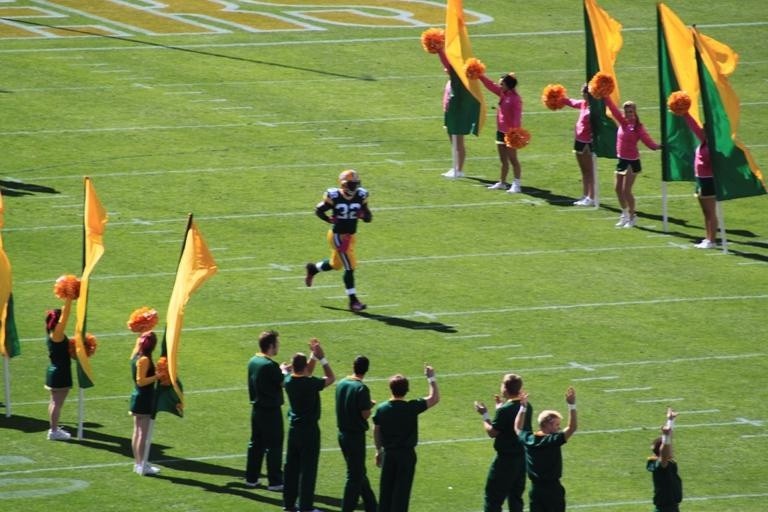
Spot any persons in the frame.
[45,309,72,440]
[602,95,664,227]
[513,386,578,511]
[305,170,374,311]
[669,105,720,249]
[245,329,292,491]
[474,373,534,512]
[281,337,336,512]
[372,361,440,512]
[560,84,596,208]
[646,406,682,512]
[334,355,377,512]
[436,48,465,178]
[128,331,165,475]
[476,71,523,193]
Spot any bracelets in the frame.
[319,358,328,367]
[309,353,318,361]
[665,419,674,429]
[482,413,489,421]
[427,376,436,383]
[567,403,577,410]
[520,406,527,413]
[661,435,673,445]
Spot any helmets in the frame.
[337,169,360,187]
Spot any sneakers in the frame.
[132,459,161,476]
[266,482,284,491]
[573,193,594,207]
[304,263,315,287]
[46,424,73,442]
[615,210,639,229]
[346,300,367,310]
[245,476,262,487]
[694,236,718,250]
[505,181,523,193]
[441,167,465,178]
[488,180,507,191]
